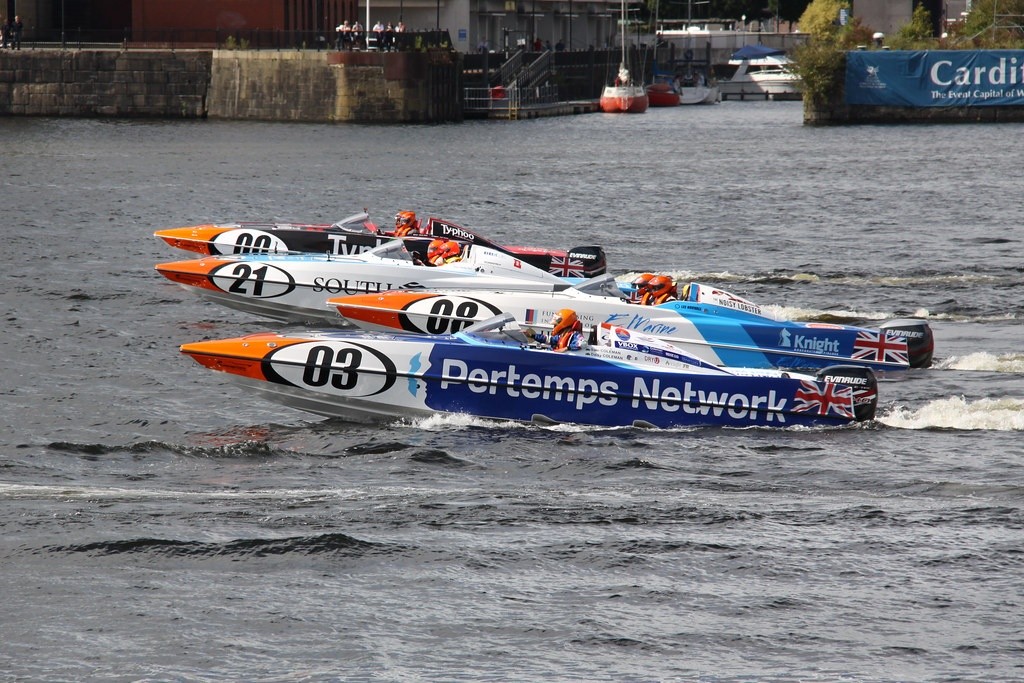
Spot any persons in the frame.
[385,21,393,52]
[336,20,350,51]
[395,21,406,52]
[377,211,419,238]
[352,20,363,52]
[414,31,709,95]
[10,16,23,49]
[623,273,675,306]
[0,19,9,49]
[520,309,588,353]
[412,240,462,266]
[373,21,384,53]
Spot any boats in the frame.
[150,211,937,435]
[716,42,807,101]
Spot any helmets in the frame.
[396,211,405,227]
[401,212,415,227]
[633,274,655,294]
[649,275,671,296]
[440,242,460,258]
[427,240,444,257]
[550,309,576,335]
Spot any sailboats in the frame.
[598,0,720,113]
